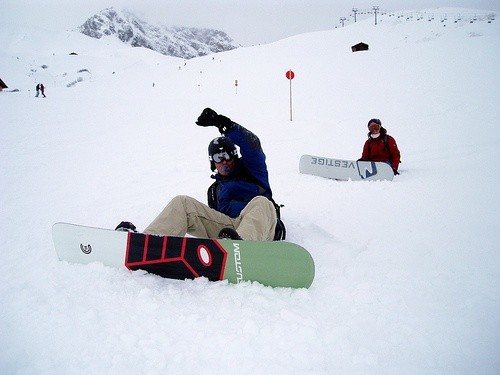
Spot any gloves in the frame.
[196,108,232,134]
[393,169,399,175]
[357,158,364,161]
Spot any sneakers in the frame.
[217,228,243,240]
[114,221,137,232]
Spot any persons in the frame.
[35,83,41,97]
[41,84,46,96]
[114,106,287,241]
[357,118,401,177]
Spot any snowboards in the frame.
[51,222,316,289]
[299,154,394,181]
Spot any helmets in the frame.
[208,137,238,172]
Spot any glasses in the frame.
[369,125,379,132]
[209,148,238,163]
